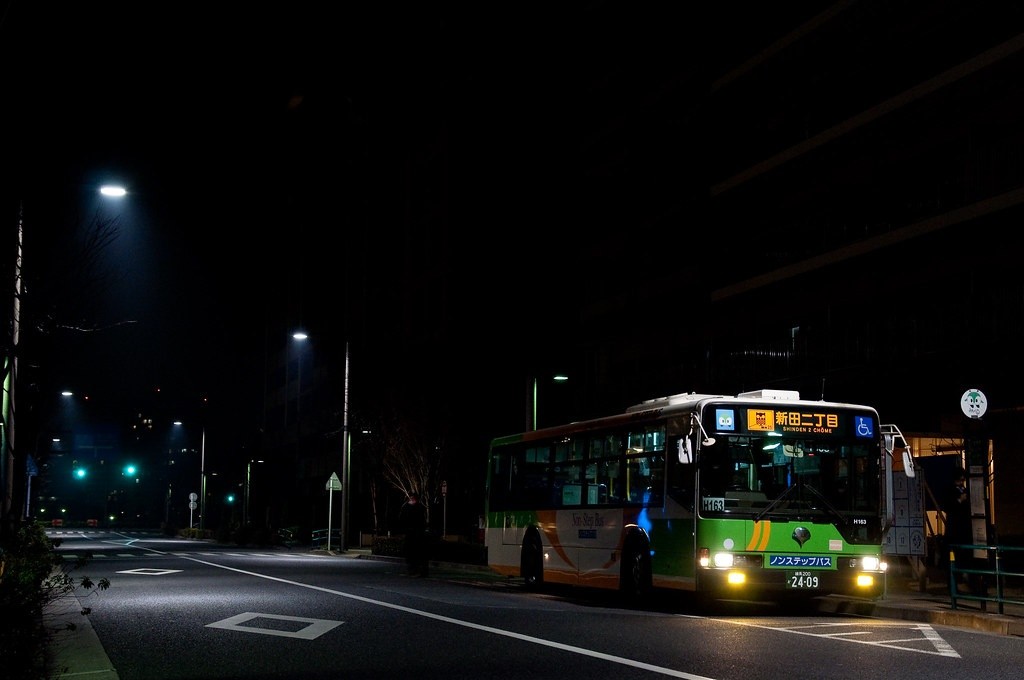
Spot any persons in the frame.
[945,467,970,594]
[398,491,432,579]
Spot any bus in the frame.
[484,389,915,617]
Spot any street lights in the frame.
[533,374,569,432]
[174,420,206,530]
[4,180,132,515]
[20,390,75,521]
[283,330,310,471]
[227,496,235,523]
